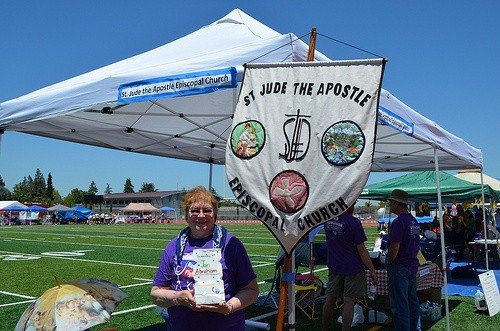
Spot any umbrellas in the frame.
[13,274,130,331]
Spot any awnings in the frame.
[452,172,500,190]
[357,168,495,316]
[122,202,177,223]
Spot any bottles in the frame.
[488,228,494,239]
[369,277,372,290]
[475,289,487,310]
[368,285,377,299]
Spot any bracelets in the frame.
[226,301,233,312]
[172,291,180,306]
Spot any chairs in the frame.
[420,241,441,263]
[262,242,317,320]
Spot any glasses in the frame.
[188,209,214,215]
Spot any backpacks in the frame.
[447,265,480,286]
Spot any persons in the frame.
[0,212,170,225]
[323,189,500,331]
[151,186,259,331]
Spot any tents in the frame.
[0,8,485,331]
[0,200,91,225]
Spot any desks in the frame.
[354,264,450,309]
[469,239,500,261]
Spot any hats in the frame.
[387,189,413,205]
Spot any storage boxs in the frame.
[419,302,443,321]
